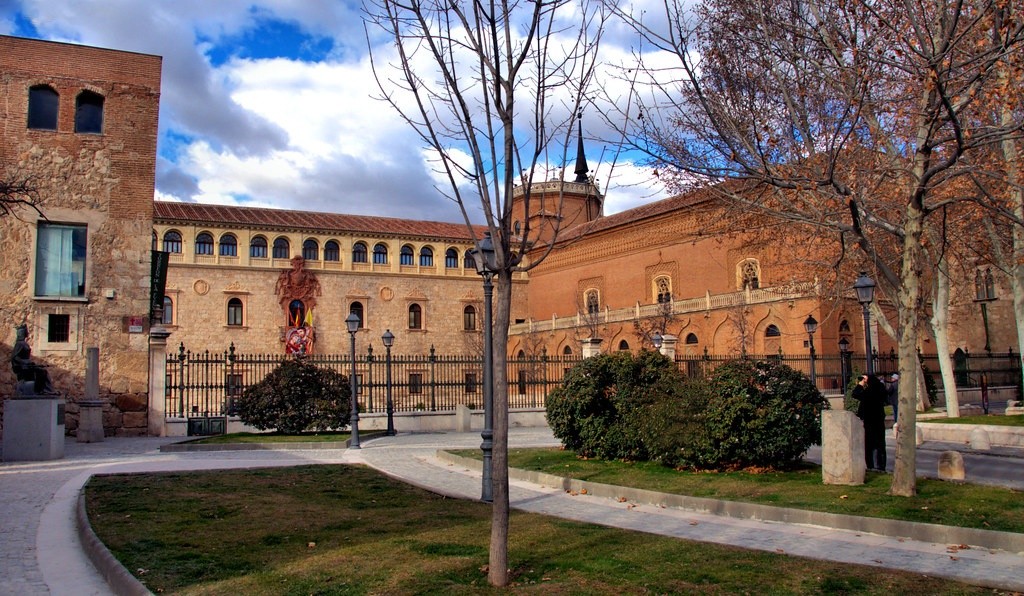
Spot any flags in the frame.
[288,310,294,324]
[294,308,301,329]
[304,306,314,327]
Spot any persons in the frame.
[888,374,898,424]
[851,372,888,472]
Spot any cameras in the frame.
[858,376,864,381]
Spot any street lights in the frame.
[344,310,362,452]
[802,313,819,386]
[469,232,507,504]
[382,329,396,437]
[852,272,877,375]
[837,337,851,396]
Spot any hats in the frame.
[890,373,898,380]
